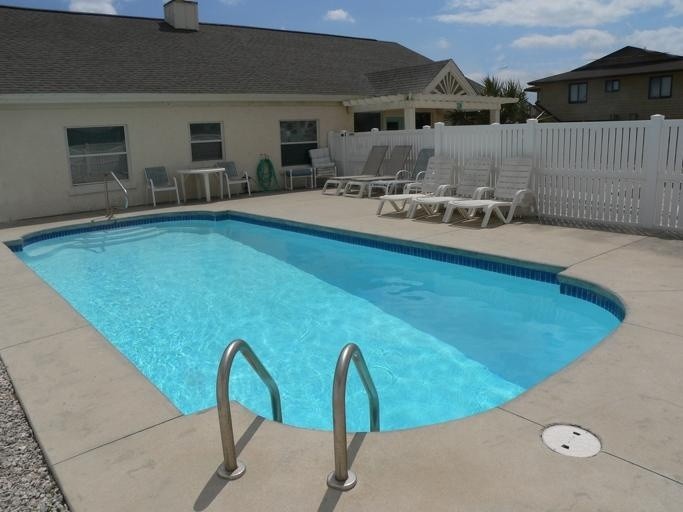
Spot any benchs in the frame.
[282,167,313,192]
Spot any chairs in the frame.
[142,165,180,207]
[214,162,252,199]
[321,144,541,229]
[308,148,337,188]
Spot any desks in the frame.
[176,167,225,202]
